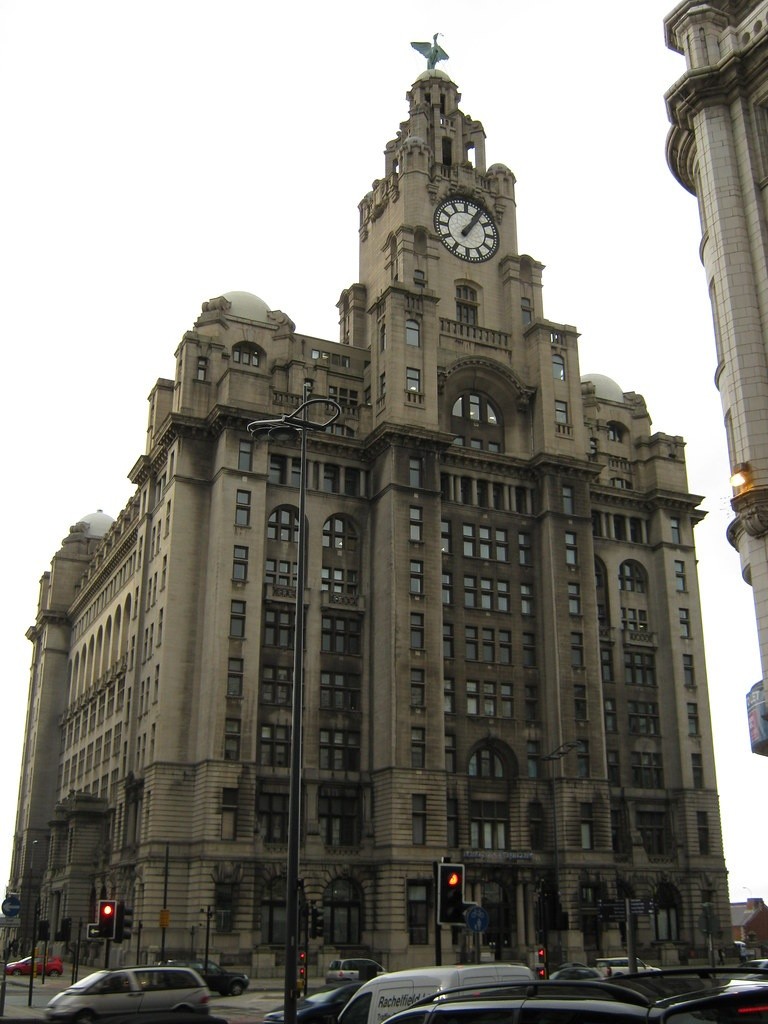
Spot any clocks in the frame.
[432,195,499,263]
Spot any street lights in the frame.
[248,398,342,1023]
[743,887,752,896]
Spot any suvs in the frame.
[157,958,248,998]
[380,966,768,1024]
[597,957,663,979]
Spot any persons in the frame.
[9,938,24,957]
[718,944,725,965]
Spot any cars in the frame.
[324,958,388,983]
[720,959,768,979]
[5,956,64,977]
[548,962,603,982]
[263,979,367,1024]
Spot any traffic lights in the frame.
[297,967,306,977]
[115,901,134,944]
[87,900,116,942]
[535,946,546,963]
[437,863,465,927]
[536,967,546,979]
[296,949,305,962]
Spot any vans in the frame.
[45,964,210,1024]
[337,963,534,1024]
